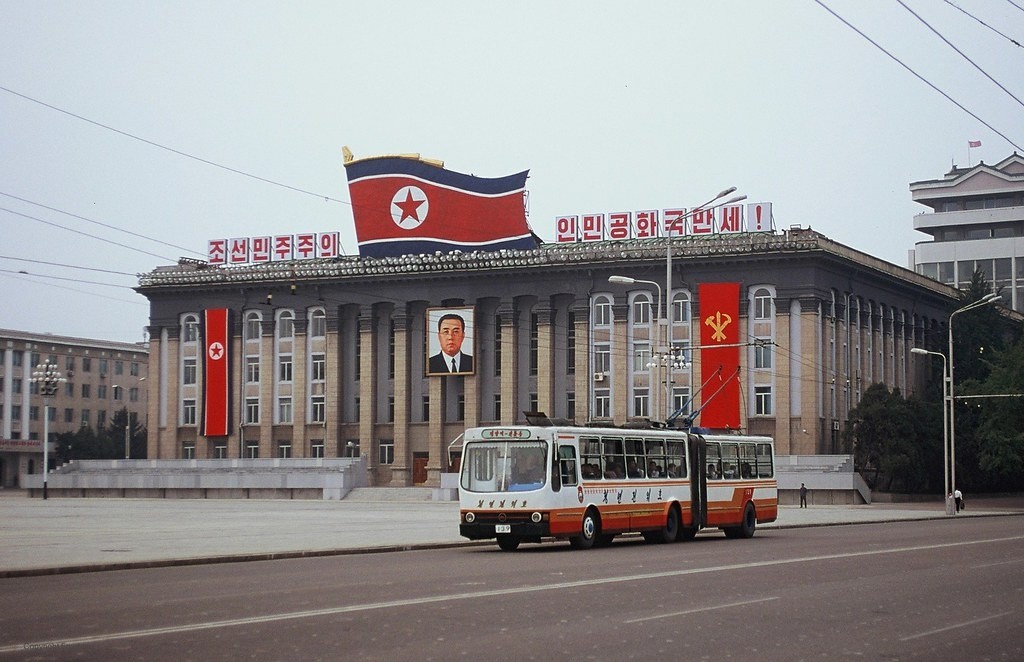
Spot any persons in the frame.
[581,461,677,481]
[429,314,473,373]
[799,483,807,508]
[707,462,752,480]
[949,488,963,513]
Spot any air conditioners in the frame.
[594,372,603,381]
[81,421,87,426]
[833,422,839,430]
[100,373,106,377]
[69,371,74,376]
[98,422,104,427]
[831,317,836,322]
[856,369,860,378]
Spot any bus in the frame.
[447,367,778,552]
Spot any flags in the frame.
[969,141,981,148]
[700,283,741,429]
[342,154,540,258]
[199,308,234,436]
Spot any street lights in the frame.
[111,376,147,458]
[26,359,68,499]
[910,292,1003,514]
[609,186,746,420]
